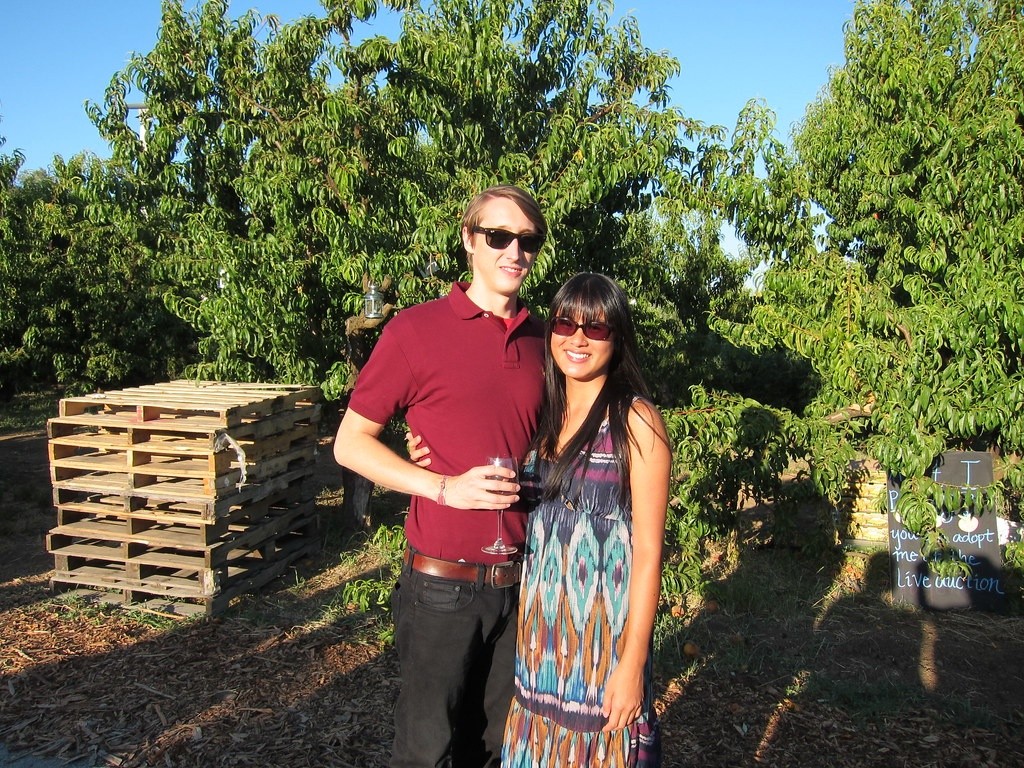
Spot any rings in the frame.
[438,475,447,506]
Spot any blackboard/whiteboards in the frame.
[885,451,1007,613]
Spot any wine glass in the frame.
[481,456,518,555]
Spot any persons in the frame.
[405,273,670,767]
[334,186,548,768]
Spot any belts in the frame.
[404,548,522,589]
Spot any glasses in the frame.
[550,316,613,341]
[471,225,547,253]
